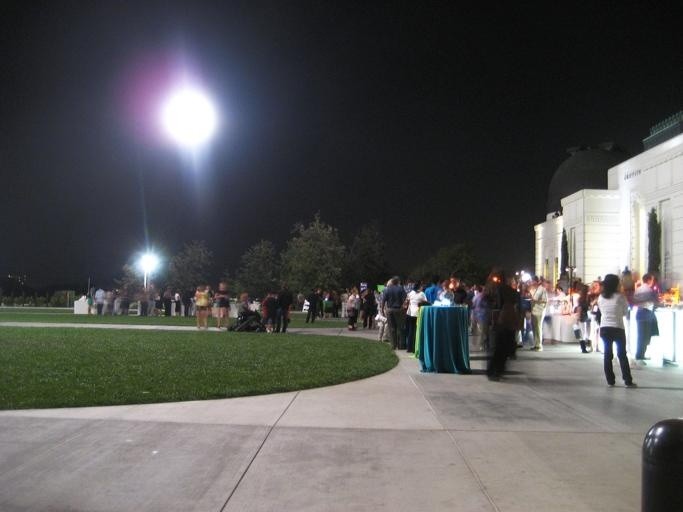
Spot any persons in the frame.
[86,281,292,334]
[380,273,552,383]
[556,274,660,366]
[597,273,637,387]
[296,282,392,343]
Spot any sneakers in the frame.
[625,383,636,388]
[608,385,614,387]
